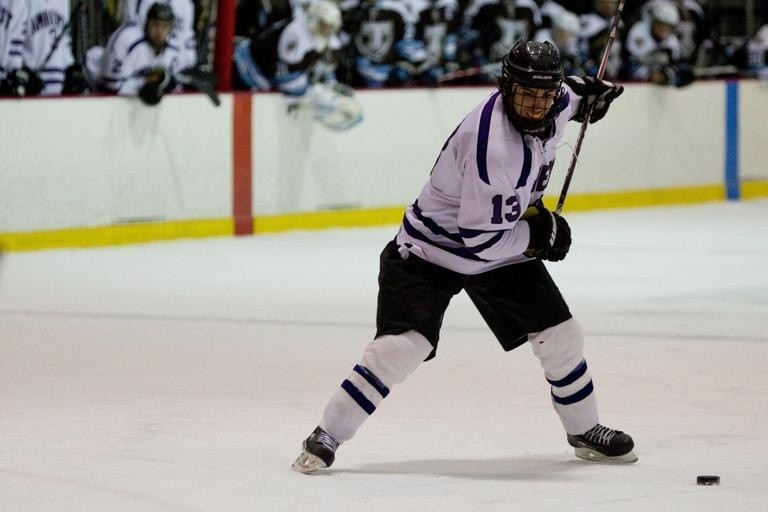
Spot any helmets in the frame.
[501,37,565,97]
[147,1,175,22]
[306,1,343,37]
[648,0,681,27]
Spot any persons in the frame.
[0,0,768,104]
[303,42,634,469]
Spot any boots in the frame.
[303,426,339,467]
[566,424,634,457]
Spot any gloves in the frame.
[662,64,692,87]
[564,75,624,125]
[140,71,176,106]
[529,211,572,263]
